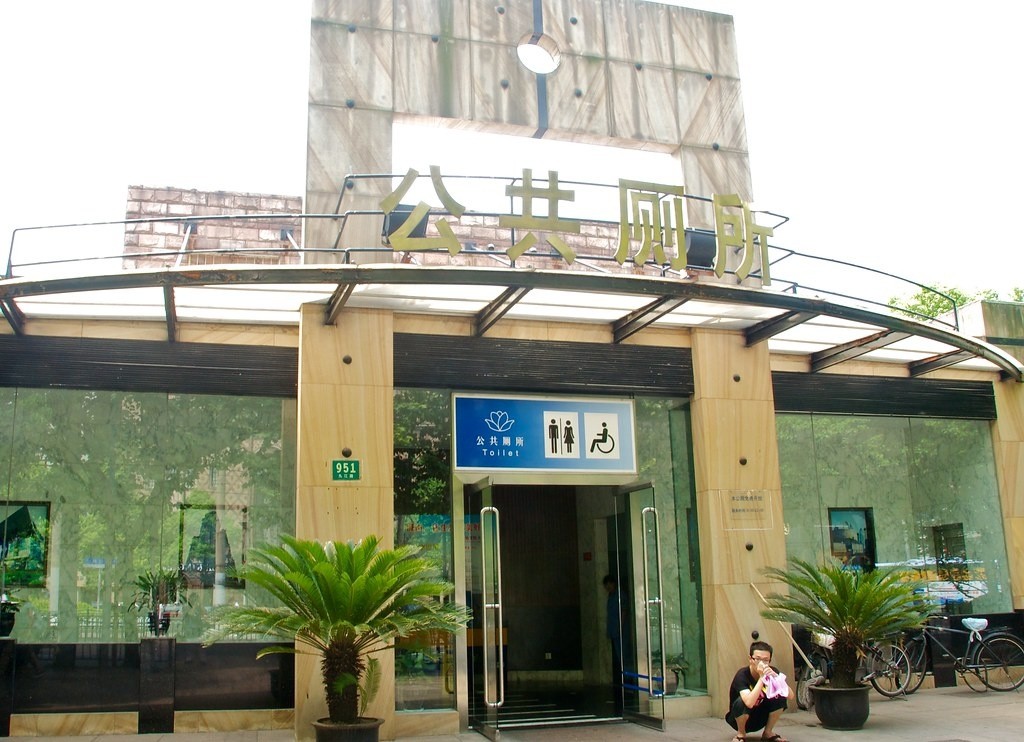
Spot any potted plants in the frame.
[757,555,945,731]
[127,568,193,637]
[200,532,474,742]
[0,588,21,636]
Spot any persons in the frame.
[603,575,629,716]
[725,641,794,742]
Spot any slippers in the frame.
[760,733,787,742]
[731,735,747,742]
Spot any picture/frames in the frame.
[0,501,51,588]
[179,503,247,590]
[828,508,872,571]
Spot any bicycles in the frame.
[894,613,1024,697]
[795,621,912,713]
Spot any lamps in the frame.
[382,203,429,249]
[684,227,716,267]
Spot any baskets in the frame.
[810,622,836,651]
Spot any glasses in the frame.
[750,656,770,665]
